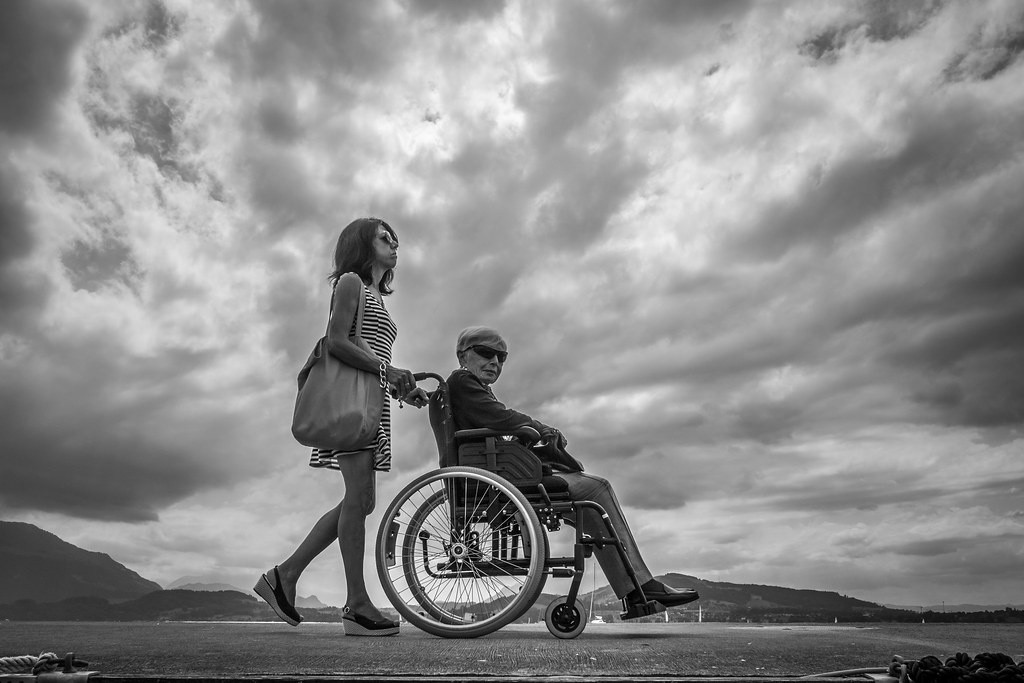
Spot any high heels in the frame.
[253,565,304,627]
[341,605,400,637]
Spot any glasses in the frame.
[462,344,508,363]
[375,231,399,250]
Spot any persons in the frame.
[253,217,430,635]
[428,325,699,615]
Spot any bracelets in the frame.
[380,362,387,389]
[392,387,405,408]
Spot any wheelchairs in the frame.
[376,375,668,640]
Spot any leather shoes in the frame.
[627,582,700,607]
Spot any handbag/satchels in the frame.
[292,273,387,451]
